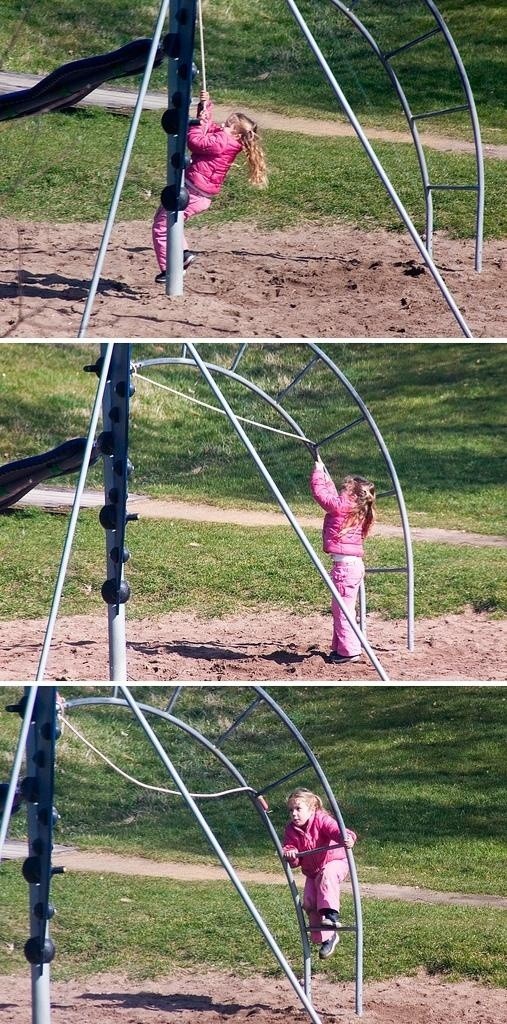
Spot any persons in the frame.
[283,786,357,959]
[152,90,271,284]
[310,454,377,663]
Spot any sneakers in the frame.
[155,271,166,283]
[184,250,195,270]
[321,910,341,928]
[330,651,358,663]
[319,932,339,959]
